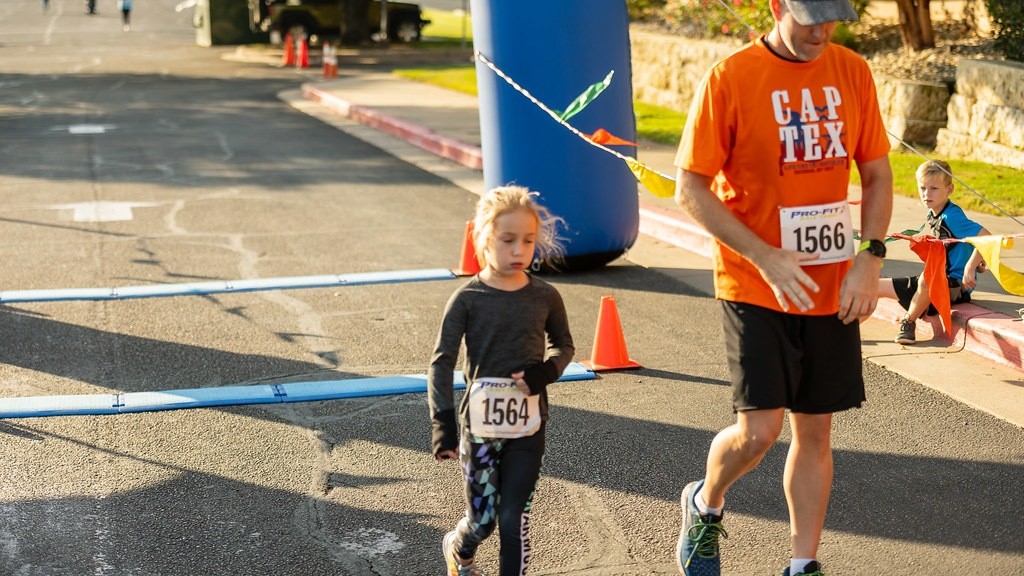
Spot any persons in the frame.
[426,185,575,576]
[857,240,887,268]
[877,161,995,347]
[673,0,891,574]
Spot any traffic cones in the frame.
[450,220,483,278]
[275,33,295,67]
[327,45,339,79]
[317,40,331,76]
[575,294,643,372]
[293,37,311,70]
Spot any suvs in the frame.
[253,0,430,45]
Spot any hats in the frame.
[785,0,857,26]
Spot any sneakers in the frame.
[442,529,476,576]
[676,479,728,576]
[895,312,916,343]
[783,559,824,576]
